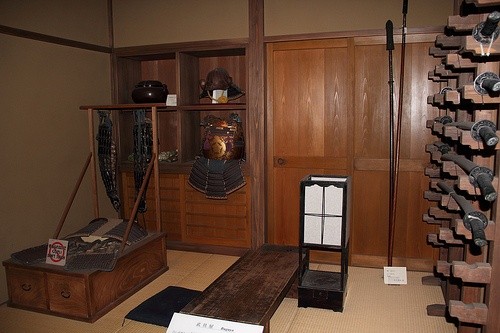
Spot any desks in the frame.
[179,249,304,333]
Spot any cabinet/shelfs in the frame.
[110,38,256,254]
[422,0,500,333]
[3,229,168,323]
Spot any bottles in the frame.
[131,80,168,103]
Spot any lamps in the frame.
[297,173,352,313]
